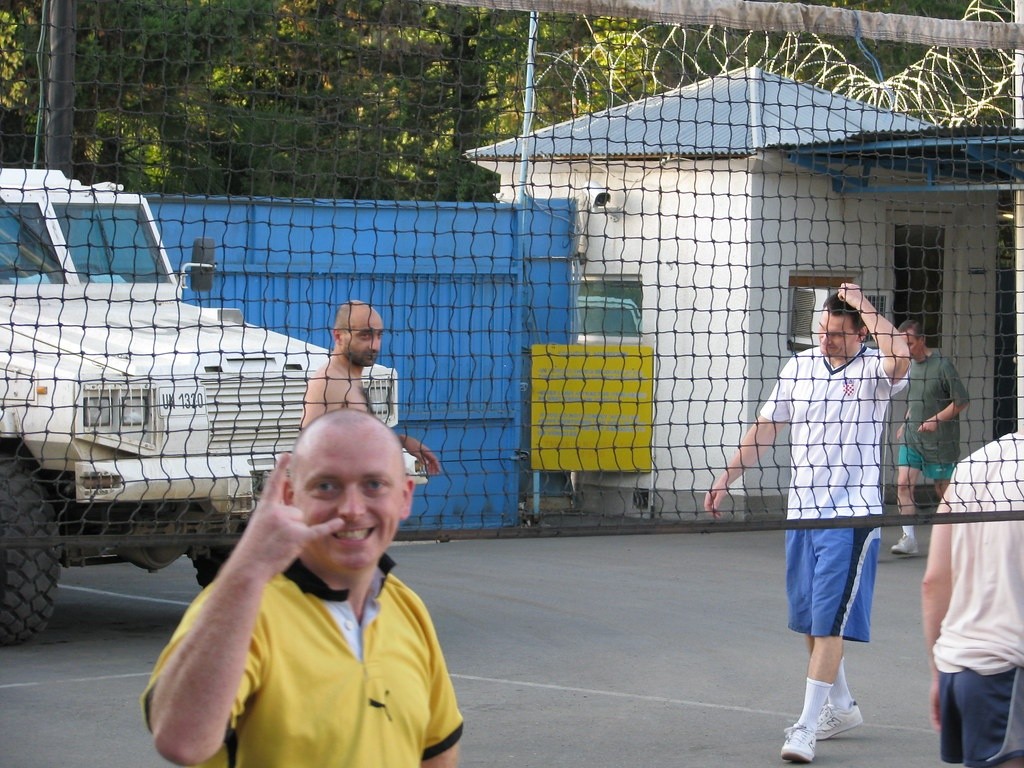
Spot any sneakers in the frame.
[781,723,817,762]
[891,533,919,554]
[816,700,863,740]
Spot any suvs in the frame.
[0,167,402,645]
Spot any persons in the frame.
[922,429,1024,768]
[889,321,969,555]
[142,409,467,767]
[705,278,912,763]
[299,299,441,482]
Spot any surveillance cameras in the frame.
[582,181,611,207]
